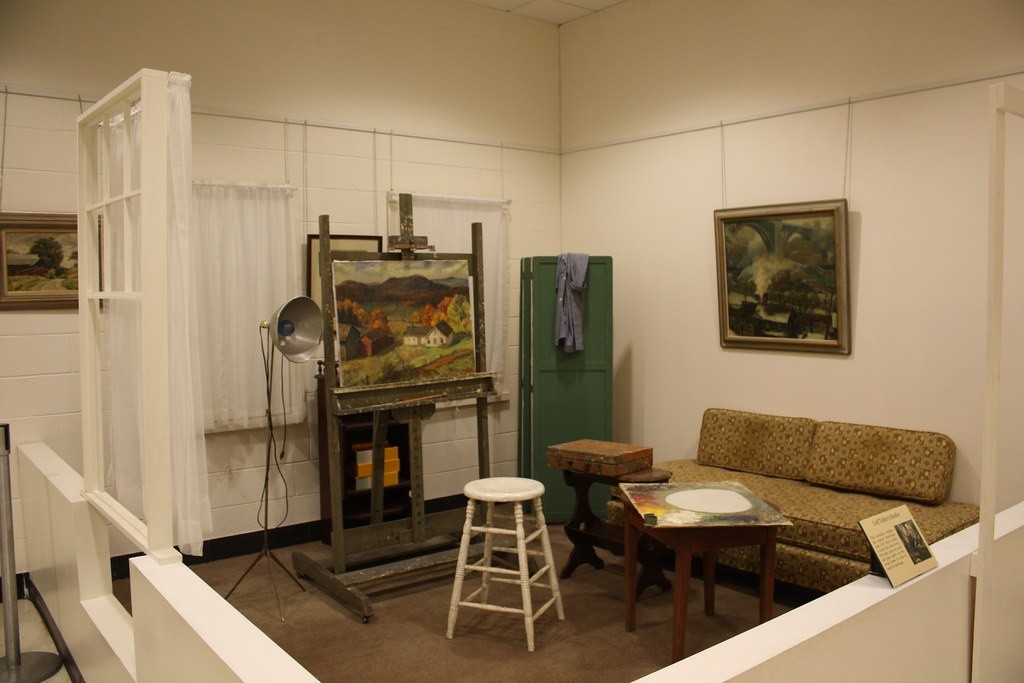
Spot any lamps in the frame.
[225,296,324,600]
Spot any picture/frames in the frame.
[715,198,850,357]
[307,234,382,315]
[0,211,104,309]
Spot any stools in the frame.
[445,476,564,652]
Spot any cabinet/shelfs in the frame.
[313,372,412,546]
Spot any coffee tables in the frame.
[611,483,780,665]
[566,469,675,601]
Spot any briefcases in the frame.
[548,439,653,476]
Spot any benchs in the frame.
[607,408,980,593]
